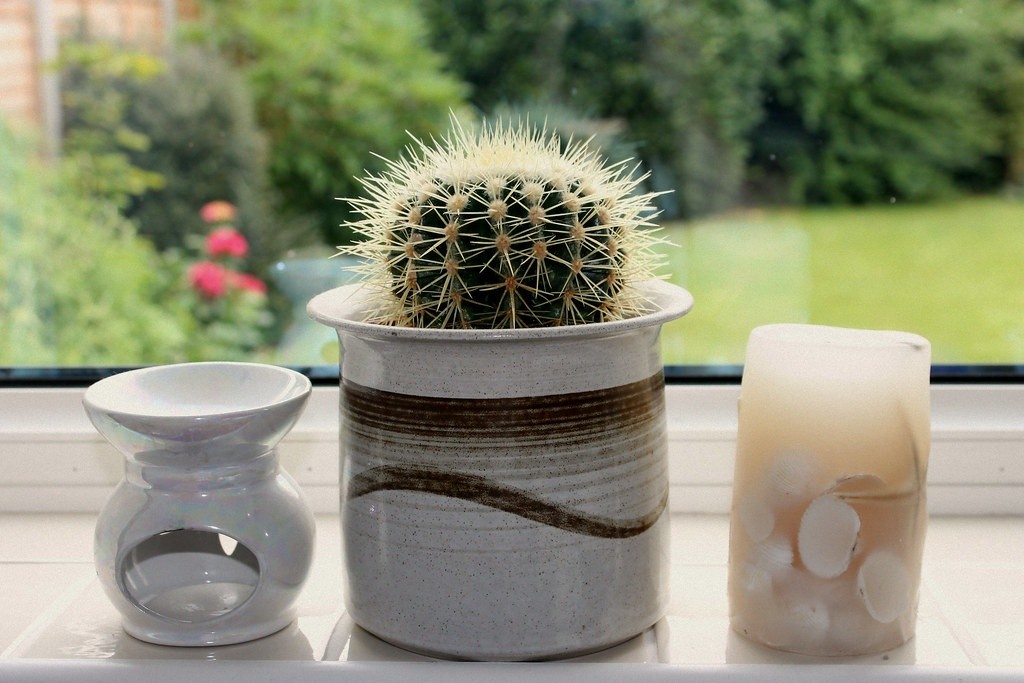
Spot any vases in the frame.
[84,363,314,651]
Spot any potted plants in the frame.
[306,105,694,658]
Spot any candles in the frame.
[726,318,932,658]
